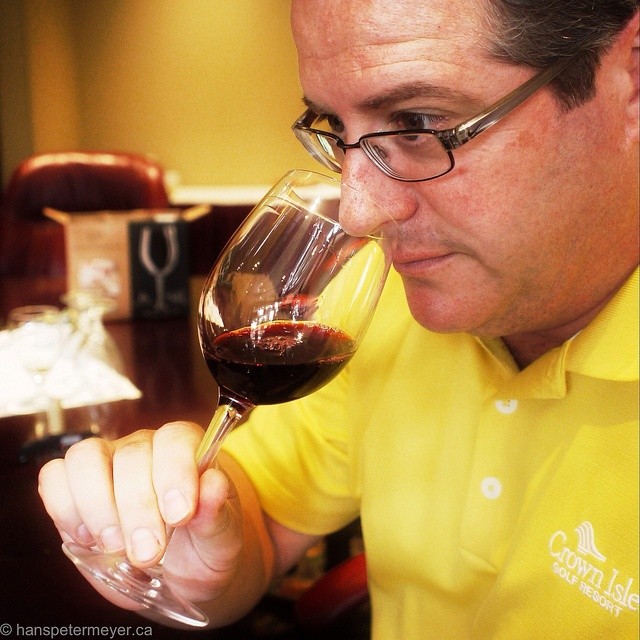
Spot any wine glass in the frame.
[60,168,400,630]
[9,306,68,437]
[139,226,179,315]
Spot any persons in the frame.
[36,0,640,637]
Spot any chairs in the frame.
[4,150,169,325]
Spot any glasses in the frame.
[291,39,588,182]
